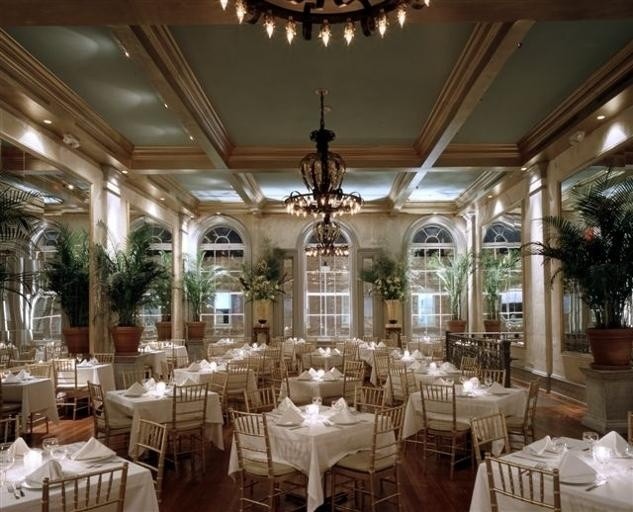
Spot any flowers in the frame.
[234,253,293,304]
[354,253,418,300]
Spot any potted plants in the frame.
[142,245,171,342]
[426,250,477,335]
[506,153,631,372]
[174,266,221,342]
[96,232,173,355]
[34,214,89,355]
[477,247,518,336]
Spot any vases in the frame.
[251,299,272,322]
[380,301,404,328]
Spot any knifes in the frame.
[286,426,306,430]
[514,455,545,463]
[585,479,607,492]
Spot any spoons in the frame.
[519,461,544,477]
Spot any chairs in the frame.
[0,333,631,512]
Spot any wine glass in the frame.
[438,376,491,397]
[0,438,16,492]
[76,353,82,364]
[3,370,10,383]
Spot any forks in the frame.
[322,420,343,429]
[6,478,24,500]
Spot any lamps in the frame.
[279,84,365,258]
[216,0,436,46]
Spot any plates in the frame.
[491,391,508,395]
[68,450,115,462]
[271,405,362,426]
[556,474,597,486]
[21,470,77,490]
[78,365,91,368]
[123,387,201,397]
[2,380,21,384]
[532,450,558,458]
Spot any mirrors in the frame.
[0,134,93,368]
[480,199,524,344]
[557,142,630,353]
[127,203,171,342]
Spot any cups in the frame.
[311,394,321,406]
[40,437,57,454]
[552,436,568,454]
[582,432,633,473]
[50,444,66,462]
[141,379,148,385]
